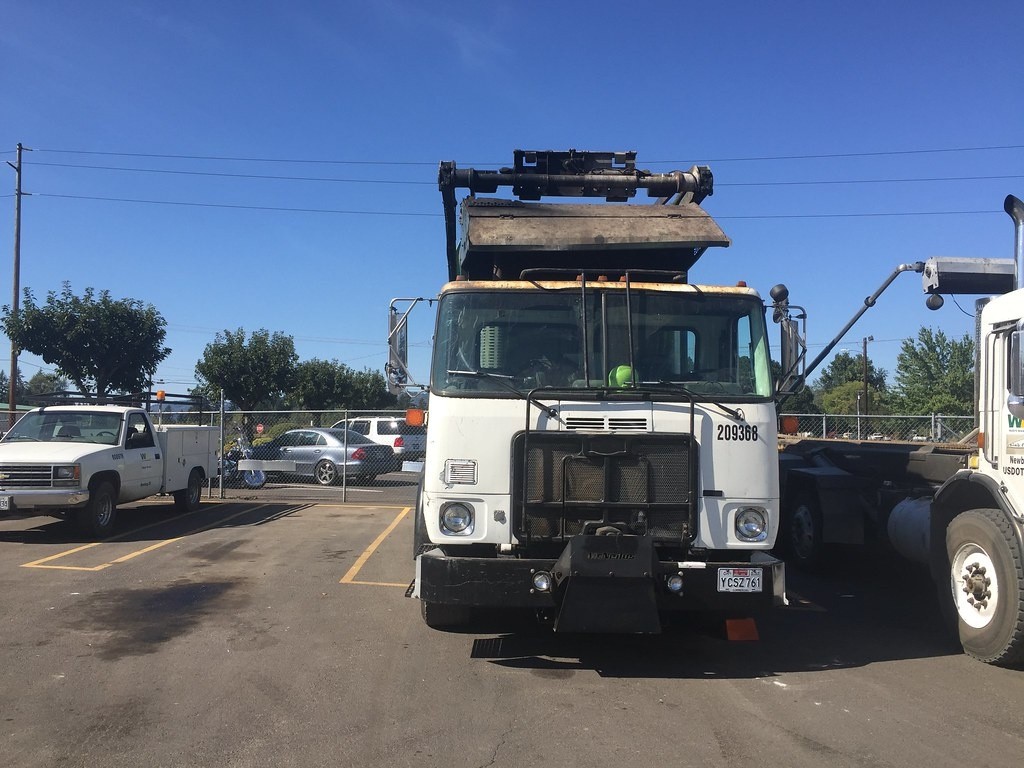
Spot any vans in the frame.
[330,416,427,470]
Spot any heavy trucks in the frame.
[770,195,1024,663]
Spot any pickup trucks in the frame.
[0,405,219,534]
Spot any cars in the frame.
[247,428,398,487]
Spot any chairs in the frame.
[58,426,81,436]
[116,426,139,439]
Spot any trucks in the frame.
[410,149,810,657]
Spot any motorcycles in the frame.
[213,426,266,489]
